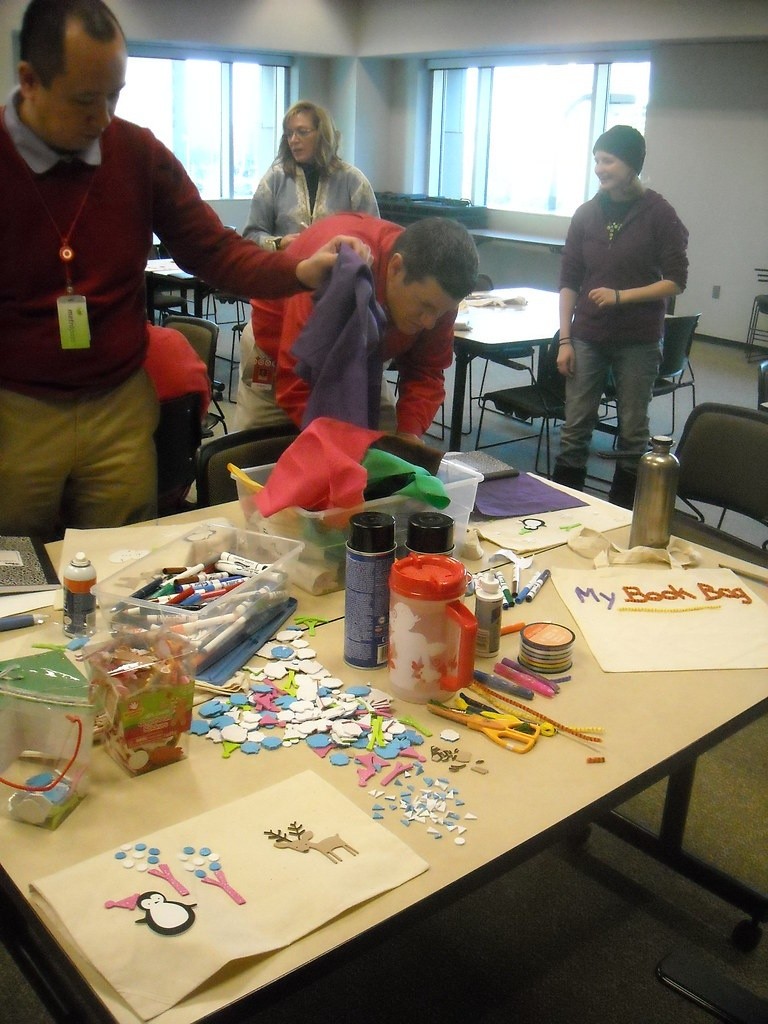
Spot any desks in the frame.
[144,257,227,403]
[0,470,768,1024]
[446,286,576,458]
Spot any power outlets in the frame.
[711,286,720,299]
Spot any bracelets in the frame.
[615,290,620,305]
[558,337,571,346]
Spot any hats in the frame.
[592,125,646,176]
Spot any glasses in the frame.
[283,128,317,138]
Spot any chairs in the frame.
[144,223,768,560]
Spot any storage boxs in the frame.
[227,433,484,593]
[88,522,306,677]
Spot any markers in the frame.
[0,614,50,632]
[493,658,560,698]
[501,623,525,637]
[109,550,290,667]
[498,585,510,610]
[472,669,534,700]
[511,563,519,597]
[515,571,540,605]
[526,569,550,602]
[496,571,514,607]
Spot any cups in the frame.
[386,554,478,705]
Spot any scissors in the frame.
[425,702,540,754]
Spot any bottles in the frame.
[627,435,681,550]
[475,569,504,659]
[405,512,457,560]
[62,552,97,639]
[342,512,398,671]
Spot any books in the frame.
[0,536,62,597]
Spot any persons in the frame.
[556,126,690,511]
[234,212,479,445]
[0,0,375,544]
[242,100,380,252]
